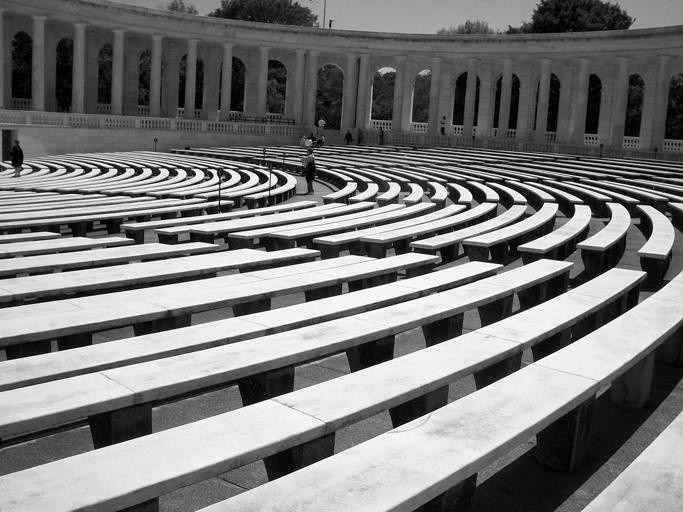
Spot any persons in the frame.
[299,133,325,147]
[440,116,446,135]
[317,117,326,130]
[343,130,352,145]
[378,127,384,145]
[8,139,23,176]
[356,129,367,144]
[301,149,315,195]
